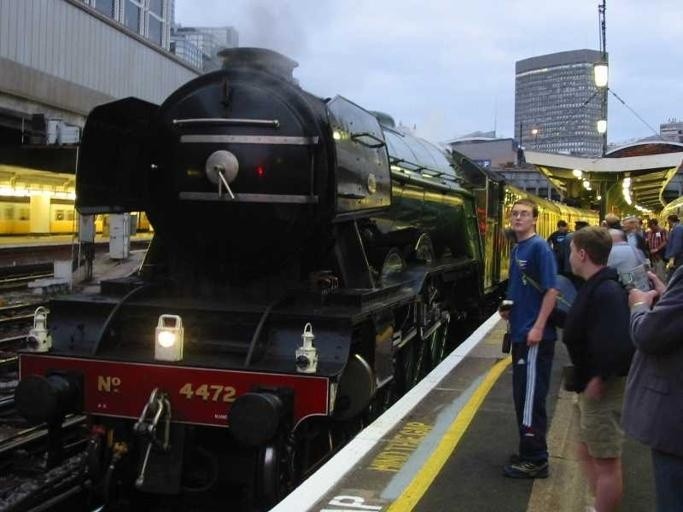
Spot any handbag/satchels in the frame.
[550,275,577,328]
[562,365,594,392]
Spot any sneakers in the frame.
[504,454,549,478]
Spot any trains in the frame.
[11,45,602,510]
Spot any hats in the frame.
[668,215,680,222]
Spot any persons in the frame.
[624,265,683,511]
[501,211,683,310]
[562,225,638,511]
[499,200,560,480]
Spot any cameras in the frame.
[500,299,515,311]
